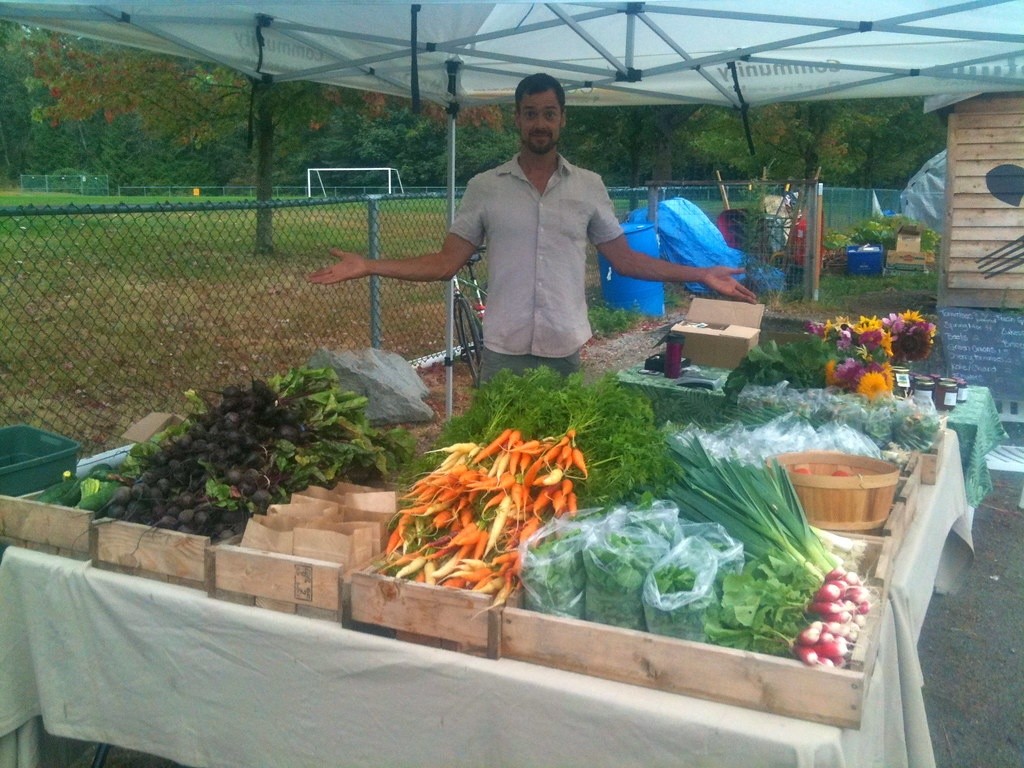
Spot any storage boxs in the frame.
[845,223,931,279]
[670,296,767,369]
[0,423,80,497]
[117,409,187,442]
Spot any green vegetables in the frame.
[520,502,745,643]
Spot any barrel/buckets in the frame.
[597,222,665,319]
[765,450,900,539]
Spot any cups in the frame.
[665,333,686,379]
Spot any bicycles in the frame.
[454,244,489,383]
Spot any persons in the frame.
[305,73,758,391]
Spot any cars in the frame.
[29,167,106,194]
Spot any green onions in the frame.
[661,432,867,576]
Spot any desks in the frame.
[0,388,996,767]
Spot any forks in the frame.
[976,235,1024,279]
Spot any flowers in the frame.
[803,310,939,397]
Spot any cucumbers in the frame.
[37,464,129,512]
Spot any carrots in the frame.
[380,428,589,612]
